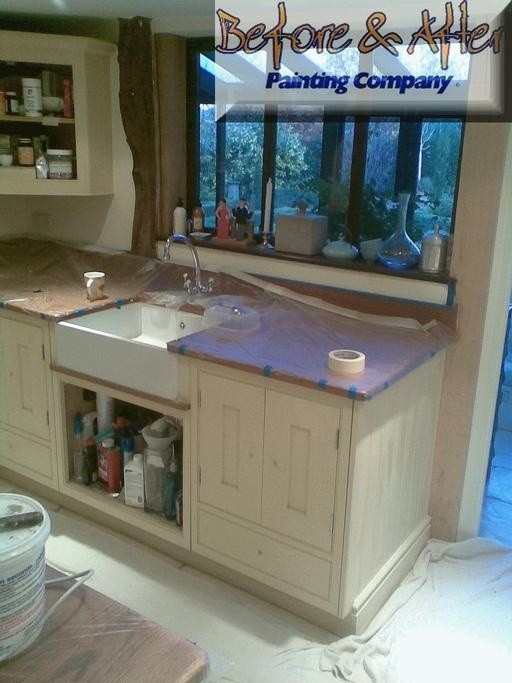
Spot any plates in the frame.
[188,230,212,241]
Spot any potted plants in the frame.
[298,172,440,263]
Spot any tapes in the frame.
[328,349,365,373]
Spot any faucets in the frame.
[163,234,206,295]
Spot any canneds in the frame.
[46,148,74,178]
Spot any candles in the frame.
[263,176,274,233]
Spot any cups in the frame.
[83,270,106,299]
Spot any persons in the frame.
[232,198,255,239]
[212,198,231,239]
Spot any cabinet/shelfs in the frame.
[0,306,59,508]
[192,347,447,637]
[0,43,115,196]
[51,368,190,568]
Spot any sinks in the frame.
[57,299,228,402]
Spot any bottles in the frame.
[419,223,447,273]
[0,69,74,118]
[1,133,73,179]
[73,411,182,527]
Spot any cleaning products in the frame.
[164,460,177,521]
[98,438,122,492]
[81,440,94,487]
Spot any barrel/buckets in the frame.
[0,491,53,662]
